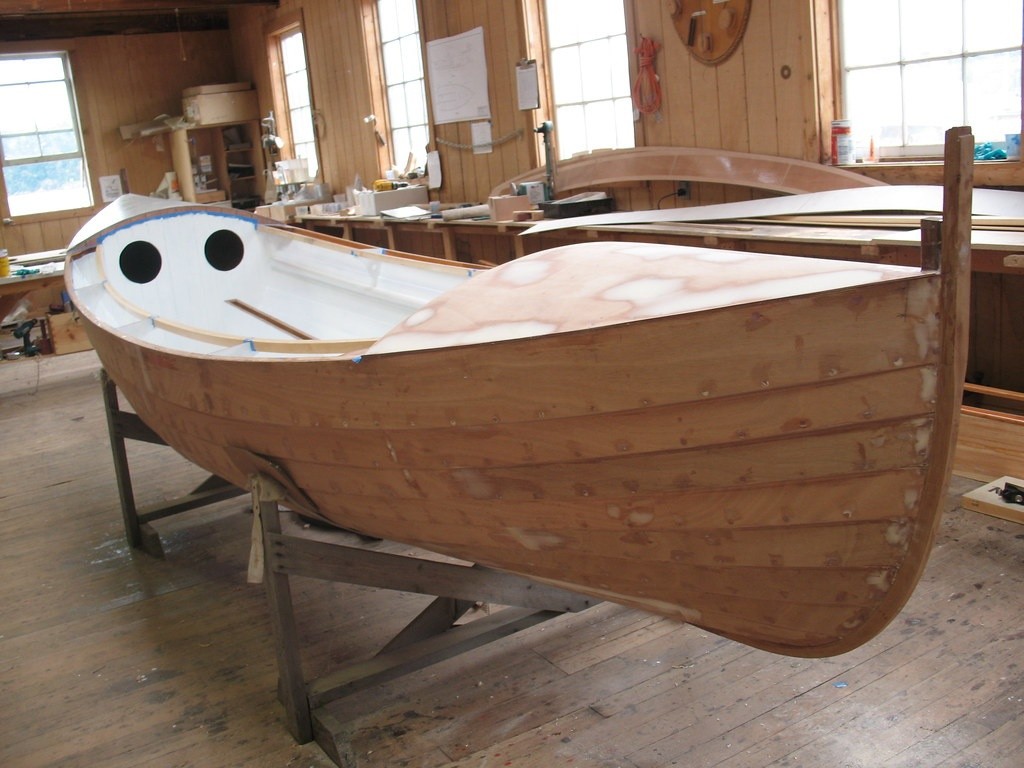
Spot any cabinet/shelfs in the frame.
[168,116,267,203]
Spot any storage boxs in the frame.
[356,186,430,216]
[44,311,95,356]
[181,81,261,125]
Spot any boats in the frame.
[63,124,977,660]
